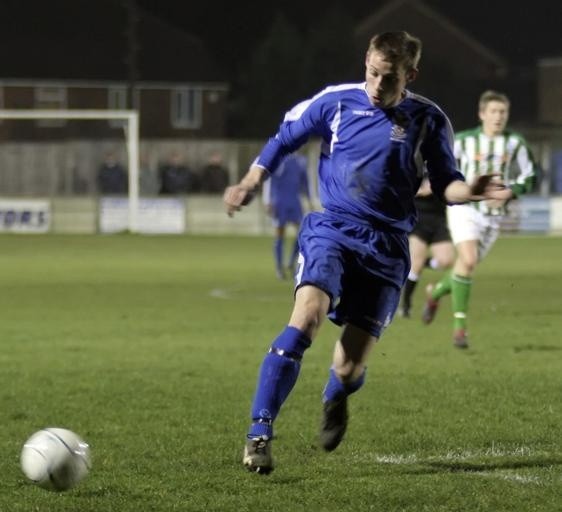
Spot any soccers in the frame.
[20,425,93,492]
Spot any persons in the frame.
[402,161,456,320]
[260,142,316,283]
[420,86,539,356]
[222,29,515,474]
[96,151,127,194]
[197,148,230,192]
[159,146,203,191]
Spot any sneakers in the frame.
[318,396,348,452]
[398,306,412,319]
[275,264,297,281]
[242,432,275,475]
[452,323,468,349]
[420,295,439,325]
